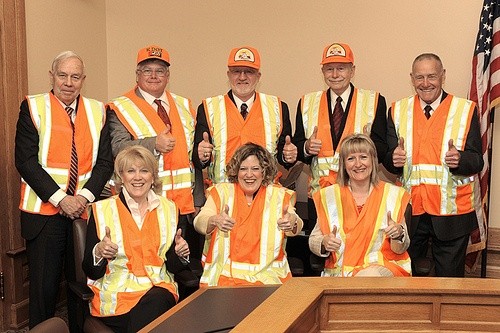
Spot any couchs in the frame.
[67,217,199,333]
[309,199,431,277]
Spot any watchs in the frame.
[399,224,404,237]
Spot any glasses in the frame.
[137,69,168,76]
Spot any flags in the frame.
[465,0,500,273]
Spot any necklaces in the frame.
[137,196,146,208]
[247,201,253,206]
[354,198,364,208]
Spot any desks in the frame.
[138,276,500,333]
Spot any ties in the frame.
[65,107,78,196]
[154,99,172,134]
[240,103,248,120]
[333,96,344,137]
[424,105,432,120]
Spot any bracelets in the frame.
[294,218,298,228]
[95,255,100,259]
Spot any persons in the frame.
[387,53,484,277]
[193,142,303,287]
[195,46,298,201]
[14,50,115,333]
[105,45,205,301]
[292,42,388,277]
[308,133,413,277]
[81,145,190,333]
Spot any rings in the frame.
[81,209,83,212]
[181,252,184,257]
[204,152,207,157]
[290,155,291,159]
[391,233,393,237]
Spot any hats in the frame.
[136,45,170,66]
[320,42,354,65]
[227,46,260,71]
